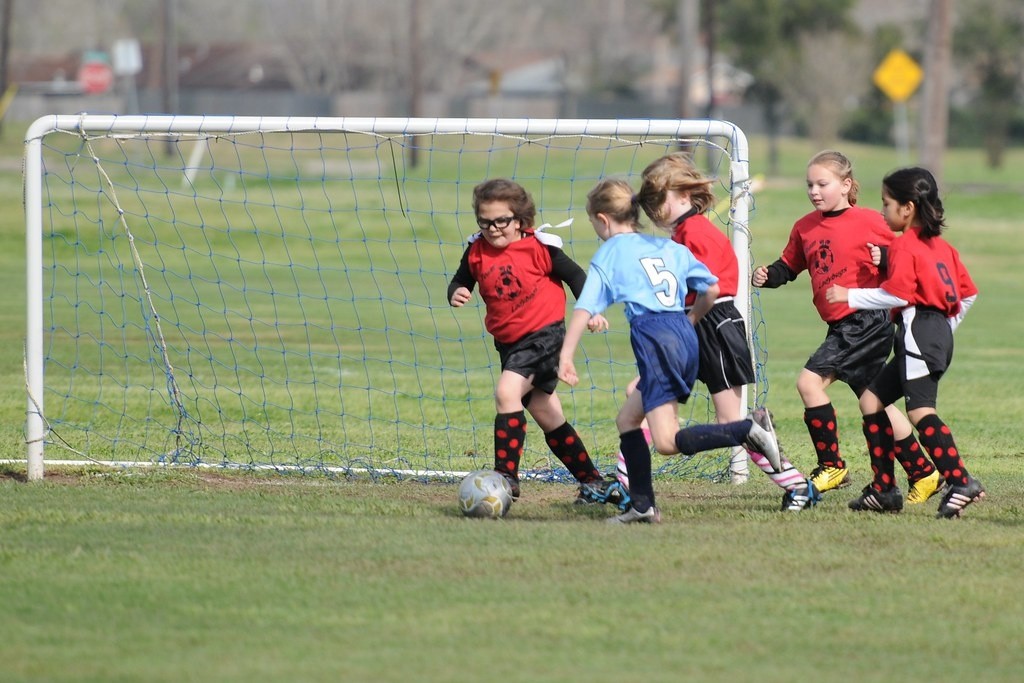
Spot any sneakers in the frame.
[906,468,947,505]
[494,468,520,500]
[808,460,852,493]
[780,478,822,513]
[606,505,660,525]
[573,473,632,513]
[847,482,904,514]
[935,474,985,519]
[746,406,784,473]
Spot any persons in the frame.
[826,167,986,519]
[557,180,785,525]
[752,149,946,505]
[618,153,820,513]
[448,180,608,505]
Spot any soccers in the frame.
[459,470,513,521]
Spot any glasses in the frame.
[477,216,517,230]
